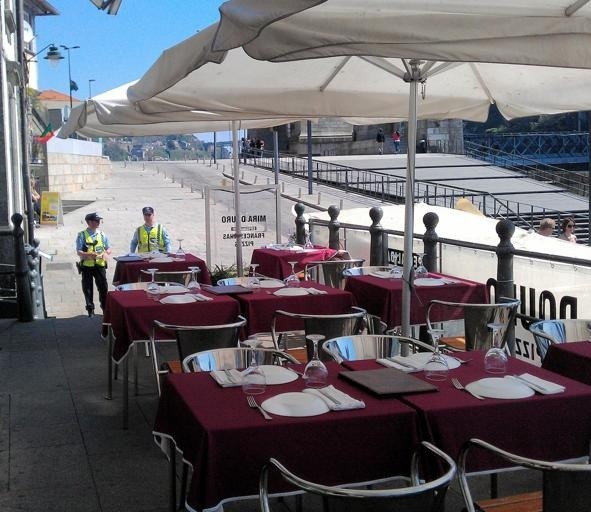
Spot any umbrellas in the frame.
[128,0,591,357]
[58,80,402,277]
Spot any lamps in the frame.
[26,43,64,69]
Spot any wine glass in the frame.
[422,329,450,383]
[484,322,508,373]
[301,332,331,387]
[285,231,314,249]
[144,250,428,301]
[173,238,187,257]
[241,339,264,396]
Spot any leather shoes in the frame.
[88,309,95,319]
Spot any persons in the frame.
[76,212,111,319]
[130,207,171,256]
[376,128,385,155]
[240,137,263,159]
[559,218,577,243]
[537,218,555,237]
[391,128,401,153]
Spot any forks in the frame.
[450,376,485,402]
[456,356,476,365]
[245,393,272,420]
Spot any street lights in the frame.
[87,78,95,99]
[58,41,81,110]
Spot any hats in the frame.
[143,207,154,215]
[85,213,104,221]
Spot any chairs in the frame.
[106,245,368,432]
[343,265,521,355]
[168,319,591,512]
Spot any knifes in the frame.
[316,386,341,406]
[224,369,237,386]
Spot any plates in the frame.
[407,351,458,371]
[241,364,299,387]
[260,392,328,417]
[466,377,537,400]
